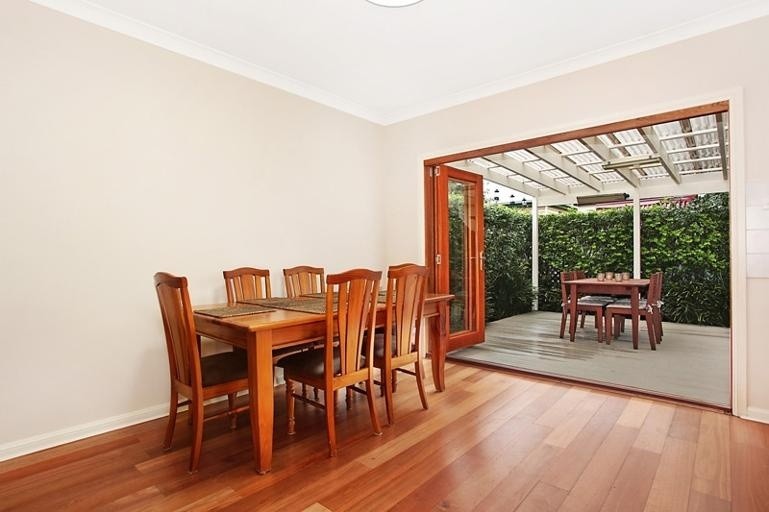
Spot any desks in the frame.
[188,290,455,475]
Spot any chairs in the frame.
[282,264,355,414]
[343,263,432,428]
[285,268,384,458]
[224,268,311,428]
[560,271,663,351]
[153,271,256,475]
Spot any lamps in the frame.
[601,154,661,170]
[365,0,425,8]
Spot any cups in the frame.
[614,273,623,282]
[622,272,630,281]
[597,273,605,281]
[605,271,614,281]
